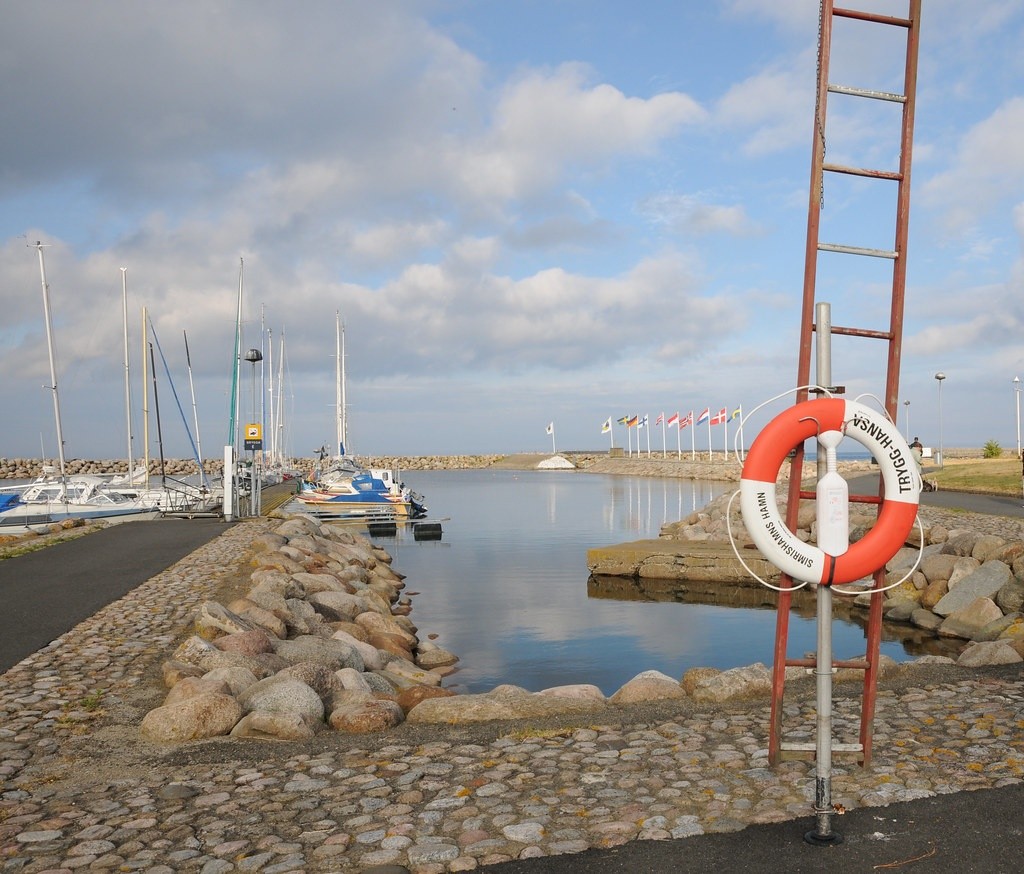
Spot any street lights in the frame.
[935,372,946,468]
[1013,376,1023,455]
[245,349,262,511]
[903,400,911,442]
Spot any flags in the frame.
[602,417,611,433]
[710,408,724,425]
[696,409,708,426]
[546,423,554,434]
[618,414,629,426]
[679,412,693,431]
[627,415,637,427]
[727,407,740,423]
[638,415,647,428]
[655,414,664,424]
[668,414,678,427]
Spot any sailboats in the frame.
[0,234,297,526]
[297,308,428,517]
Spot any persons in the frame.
[910,437,923,457]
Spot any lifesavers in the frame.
[736,395,926,591]
